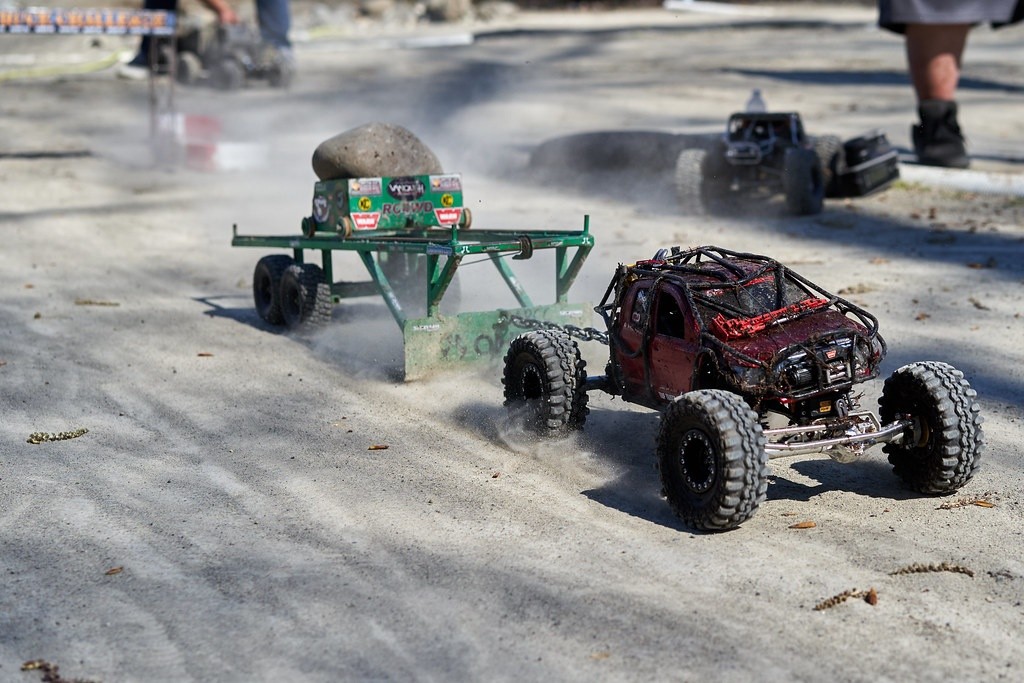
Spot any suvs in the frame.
[152,0,296,92]
[677,109,847,219]
[498,244,983,533]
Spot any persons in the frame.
[876,0,1024,170]
[114,0,294,81]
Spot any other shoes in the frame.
[913,99,970,168]
[116,46,170,80]
